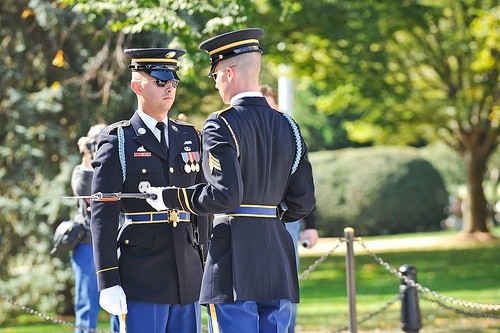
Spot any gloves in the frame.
[146,187,169,211]
[99,285,128,316]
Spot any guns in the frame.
[59,192,157,199]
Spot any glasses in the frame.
[212,65,236,81]
[131,79,178,88]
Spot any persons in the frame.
[258,84,319,271]
[70,124,109,333]
[90,47,210,333]
[145,28,316,333]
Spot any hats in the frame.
[199,28,264,77]
[124,48,185,80]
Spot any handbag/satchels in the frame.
[53,221,85,251]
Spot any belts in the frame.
[225,204,277,217]
[124,209,190,224]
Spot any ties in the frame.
[155,122,169,153]
